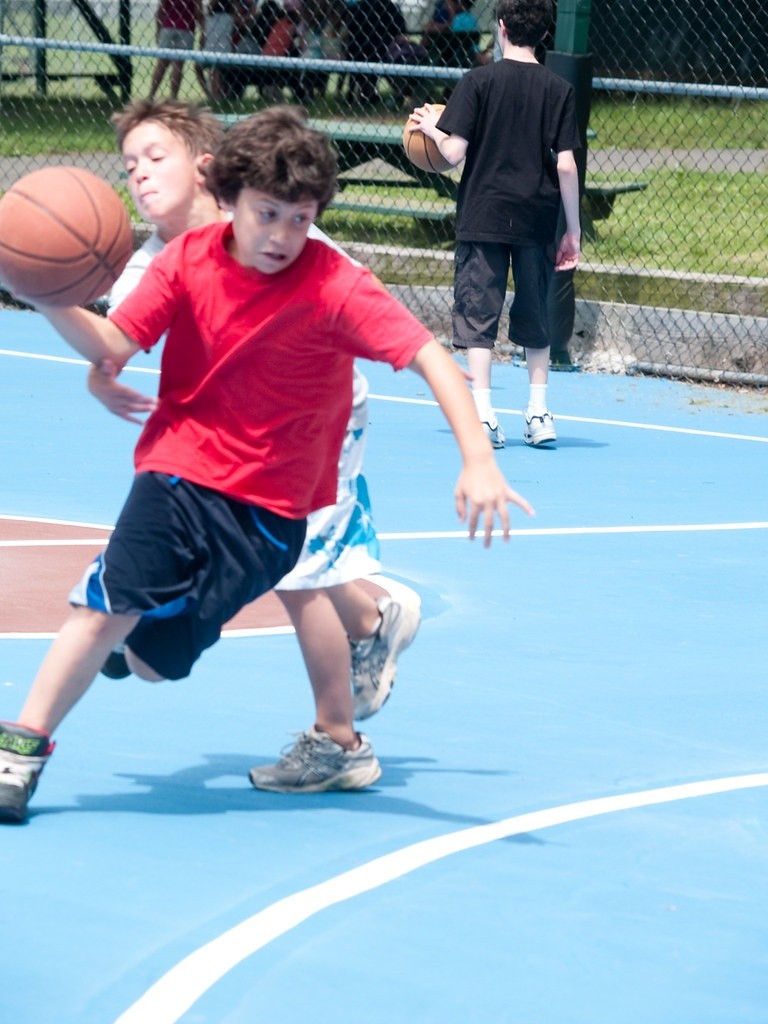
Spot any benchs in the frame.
[336,173,648,234]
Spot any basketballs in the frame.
[1,168,133,307]
[403,104,463,172]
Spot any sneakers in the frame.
[523,410,556,445]
[100,640,133,680]
[346,597,422,721]
[249,726,381,793]
[480,421,505,448]
[0,720,57,820]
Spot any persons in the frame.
[0,98,536,815]
[148,0,206,101]
[89,97,478,794]
[195,0,495,110]
[407,0,582,453]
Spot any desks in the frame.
[327,130,604,254]
